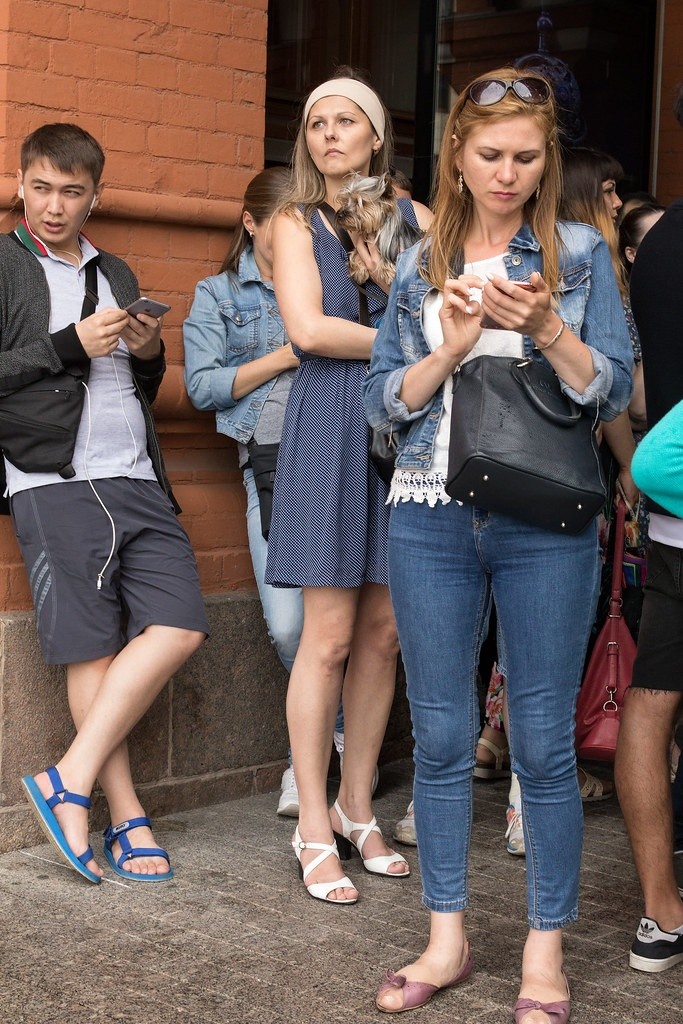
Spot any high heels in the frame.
[292,825,357,904]
[331,799,410,878]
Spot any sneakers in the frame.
[394,797,417,845]
[332,731,378,795]
[277,766,300,818]
[504,804,526,854]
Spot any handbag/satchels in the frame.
[0,363,85,479]
[444,355,606,532]
[574,614,638,756]
[248,444,280,540]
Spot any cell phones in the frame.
[126,297,170,326]
[480,281,537,330]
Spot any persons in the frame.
[394,153,667,853]
[263,69,435,906]
[0,124,209,883]
[612,200,683,972]
[183,168,380,817]
[362,68,635,1024]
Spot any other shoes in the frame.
[672,850,683,896]
[629,916,683,971]
[514,968,570,1024]
[377,942,472,1013]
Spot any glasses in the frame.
[456,76,555,126]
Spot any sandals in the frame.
[102,817,174,881]
[474,738,513,777]
[577,766,613,801]
[22,765,100,884]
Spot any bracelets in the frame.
[540,319,564,350]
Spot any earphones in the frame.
[20,186,25,199]
[89,194,96,210]
